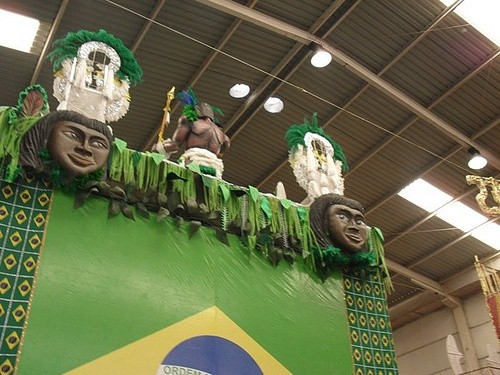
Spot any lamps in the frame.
[398,177,500,251]
[310,47,333,68]
[229,83,251,99]
[0,8,41,55]
[467,146,488,170]
[263,97,285,114]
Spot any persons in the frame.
[172,100,231,179]
[309,192,372,255]
[20,109,115,185]
